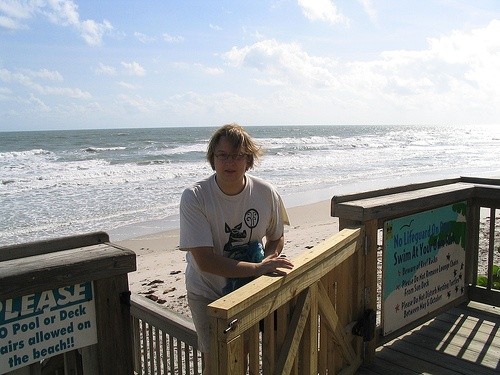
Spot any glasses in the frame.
[213,151,248,161]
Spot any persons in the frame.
[178,124,295,375]
